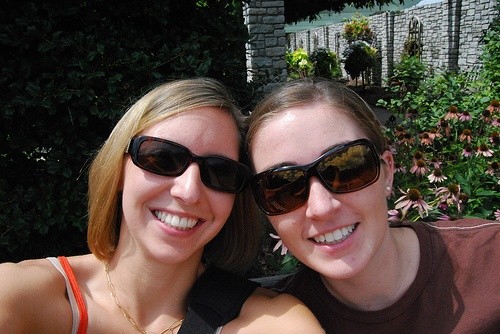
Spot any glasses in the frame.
[124,135,248,193]
[250,138,382,216]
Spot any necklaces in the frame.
[101,254,188,334]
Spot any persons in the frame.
[243,75,500,334]
[1,75,326,334]
